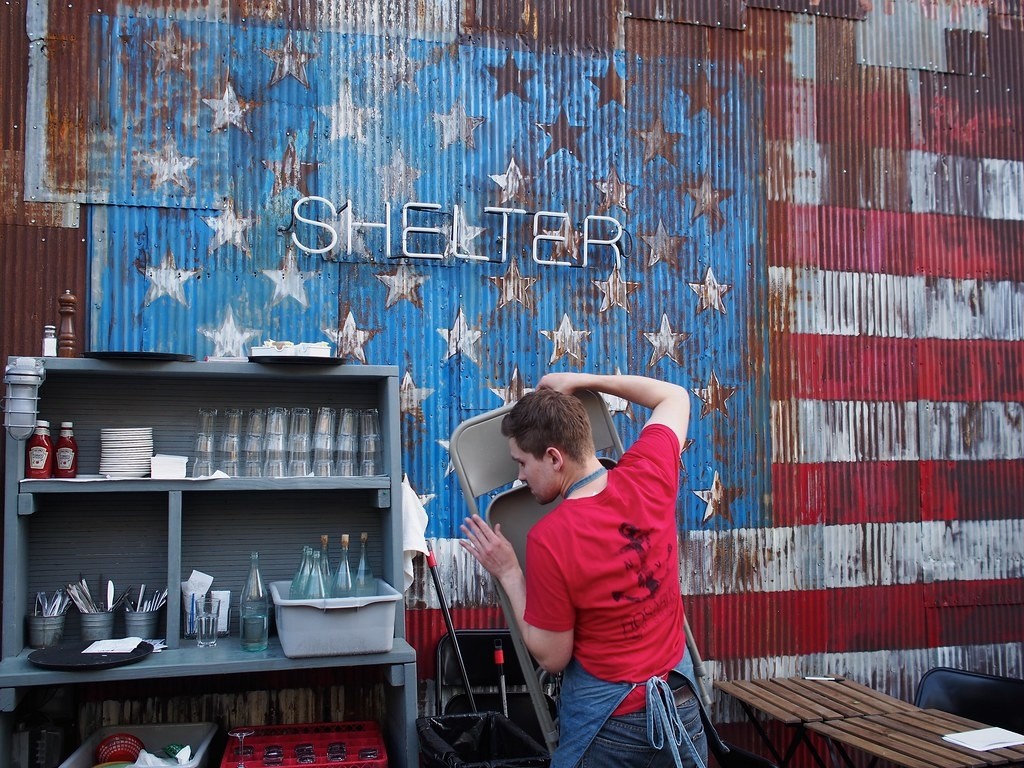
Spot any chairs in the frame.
[914,667,1024,736]
[449,390,713,759]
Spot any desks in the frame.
[711,673,922,768]
[804,708,1024,768]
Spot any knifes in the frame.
[63,572,131,614]
[34,589,72,617]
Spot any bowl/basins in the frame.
[250,345,294,356]
[294,345,331,358]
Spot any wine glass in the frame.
[228,727,255,768]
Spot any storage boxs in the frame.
[60,721,388,768]
[270,575,403,659]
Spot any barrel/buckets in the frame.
[80,613,113,640]
[26,611,66,650]
[124,611,159,640]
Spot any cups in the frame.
[192,407,382,477]
[182,594,232,648]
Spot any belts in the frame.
[630,683,695,713]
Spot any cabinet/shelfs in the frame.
[0,357,417,768]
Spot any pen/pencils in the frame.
[802,677,845,681]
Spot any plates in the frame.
[99,427,154,479]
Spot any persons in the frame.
[459,371,729,768]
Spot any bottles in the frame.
[55,422,78,477]
[289,531,375,601]
[42,325,57,357]
[239,552,270,651]
[26,420,56,478]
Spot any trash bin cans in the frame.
[416,711,551,768]
